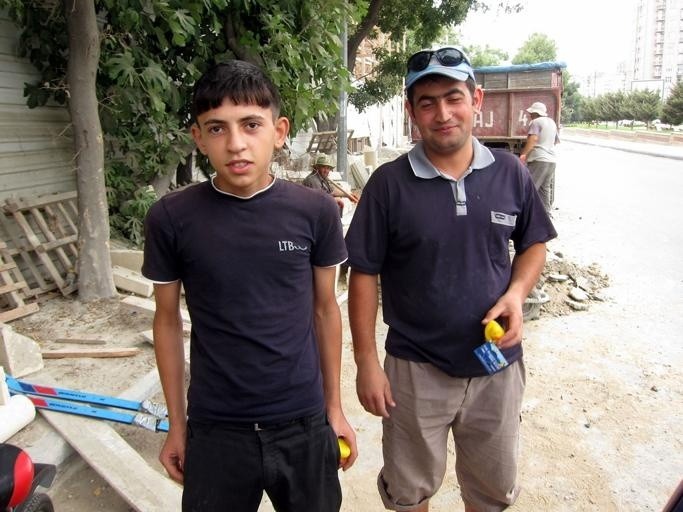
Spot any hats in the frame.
[404,47,476,90]
[527,102,548,116]
[313,157,336,170]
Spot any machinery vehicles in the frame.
[411,62,563,206]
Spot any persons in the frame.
[299,154,359,214]
[343,42,560,510]
[139,59,360,511]
[519,102,560,217]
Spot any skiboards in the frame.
[3,374,170,433]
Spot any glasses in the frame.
[407,48,470,72]
